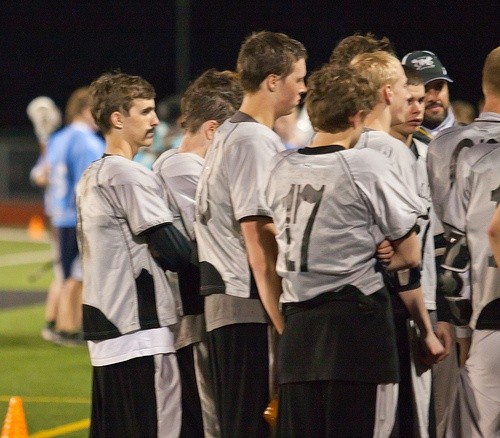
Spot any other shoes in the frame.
[41,321,53,340]
[53,331,83,347]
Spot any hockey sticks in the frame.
[27,257,61,284]
[26,96,62,158]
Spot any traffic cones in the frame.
[27,215,46,238]
[0,395,29,438]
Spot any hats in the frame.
[402,50,454,85]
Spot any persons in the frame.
[130,94,185,172]
[152,70,243,438]
[74,72,205,438]
[26,85,107,350]
[194,30,310,438]
[330,33,500,438]
[262,65,423,438]
[271,78,318,150]
